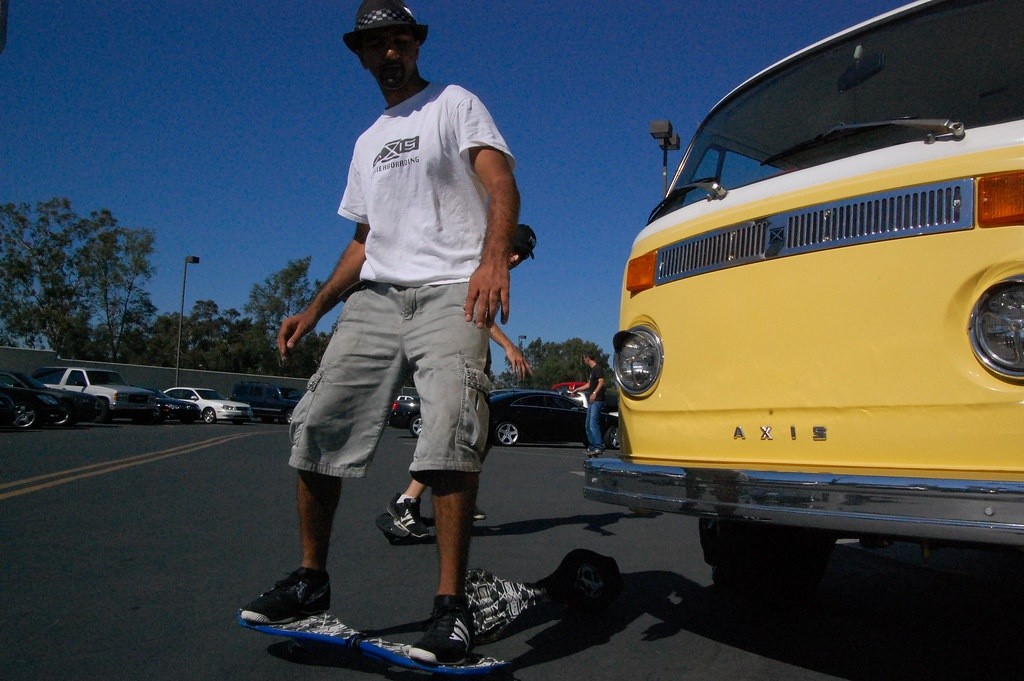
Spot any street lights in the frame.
[175,255,201,388]
[647,119,681,199]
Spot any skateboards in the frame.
[580,449,605,460]
[460,547,624,641]
[375,507,489,545]
[232,602,513,681]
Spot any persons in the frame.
[570,351,605,456]
[240,0,537,666]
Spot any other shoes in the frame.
[587,447,605,454]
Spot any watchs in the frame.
[593,392,596,394]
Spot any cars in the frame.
[391,394,496,438]
[549,382,590,397]
[135,387,201,425]
[479,390,623,450]
[163,387,255,426]
[0,382,71,433]
[397,396,413,402]
[0,368,95,428]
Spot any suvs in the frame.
[230,381,304,426]
[30,366,158,425]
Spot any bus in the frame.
[580,0,1024,615]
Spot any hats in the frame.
[514,224,536,260]
[343,0,428,53]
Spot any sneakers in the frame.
[408,597,475,665]
[473,504,486,519]
[241,567,331,623]
[386,493,430,537]
